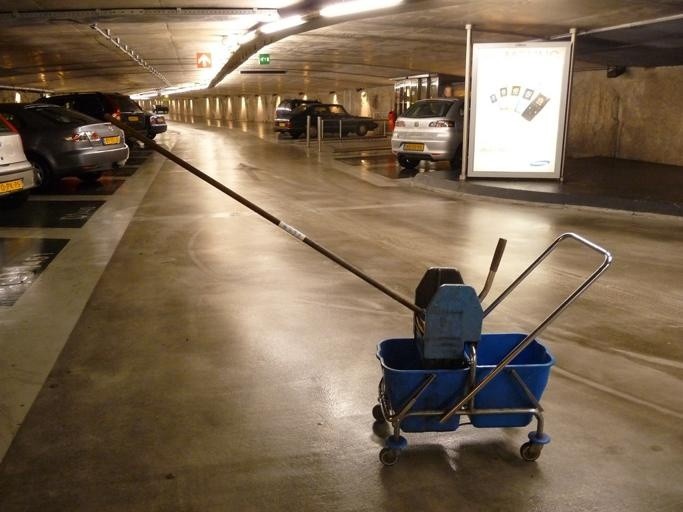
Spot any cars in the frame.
[276,102,377,140]
[274,97,325,136]
[389,96,464,173]
[2,83,169,213]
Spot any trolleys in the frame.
[371,231,612,467]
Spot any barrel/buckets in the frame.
[376,336,471,432]
[467,332,555,429]
